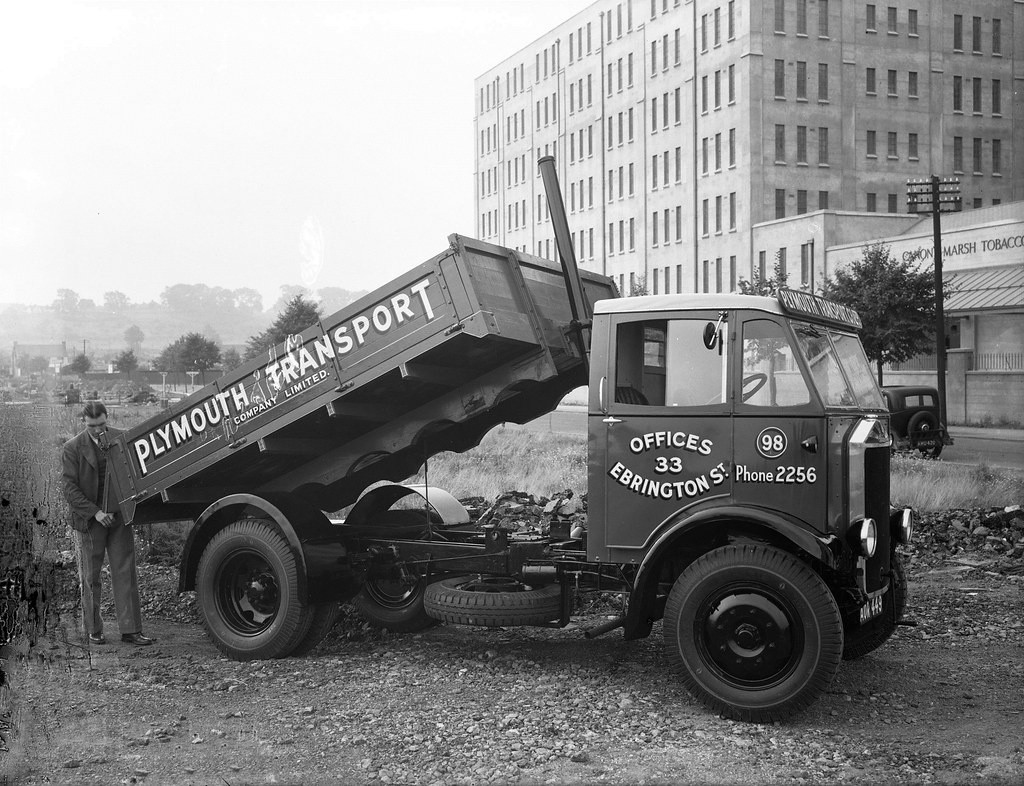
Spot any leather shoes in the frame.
[121,631,152,644]
[89,630,105,643]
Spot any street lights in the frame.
[186,371,200,392]
[158,372,169,401]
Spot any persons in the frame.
[61,401,152,645]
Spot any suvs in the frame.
[848,386,955,460]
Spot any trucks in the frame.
[97,152,919,718]
